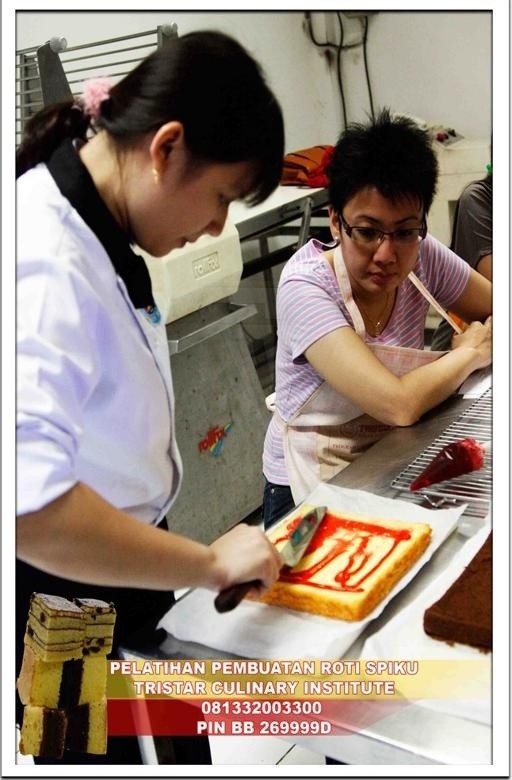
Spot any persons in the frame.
[447,169,491,284]
[259,110,491,534]
[17,28,288,765]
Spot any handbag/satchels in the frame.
[280,145,333,187]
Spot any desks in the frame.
[227,185,329,358]
[115,369,492,764]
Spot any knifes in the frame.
[208,501,329,614]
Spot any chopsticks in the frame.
[408,271,464,335]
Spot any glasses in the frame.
[340,213,427,247]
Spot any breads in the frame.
[241,505,432,621]
[424,529,493,652]
[16,592,118,760]
